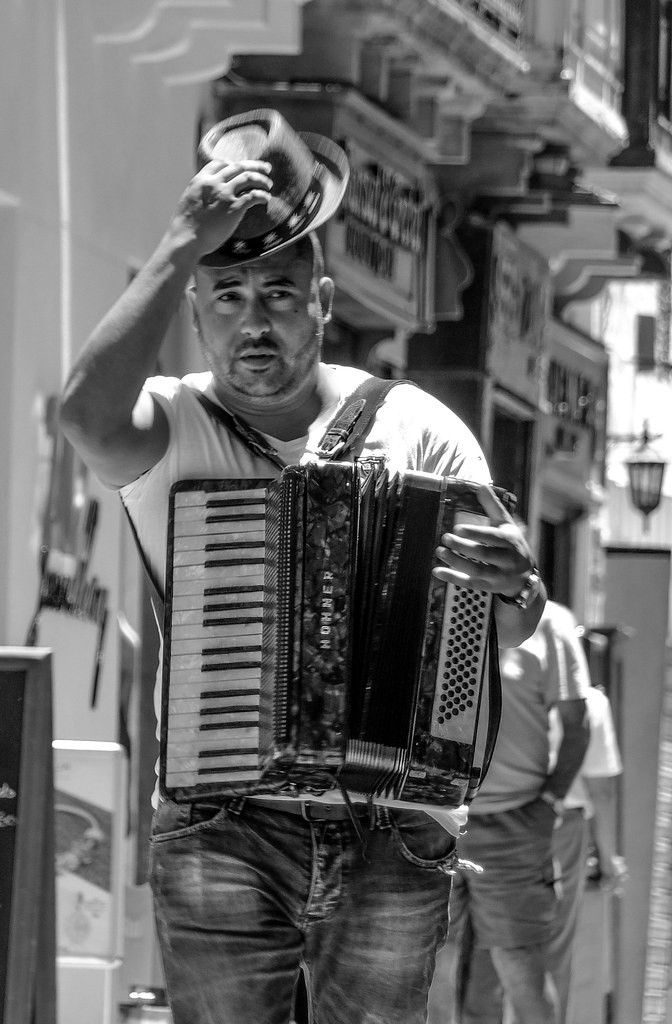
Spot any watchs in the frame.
[496,563,542,610]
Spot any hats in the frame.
[186,107,352,269]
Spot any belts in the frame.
[237,798,399,826]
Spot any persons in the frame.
[422,600,591,1024]
[453,681,630,1024]
[55,162,548,1024]
